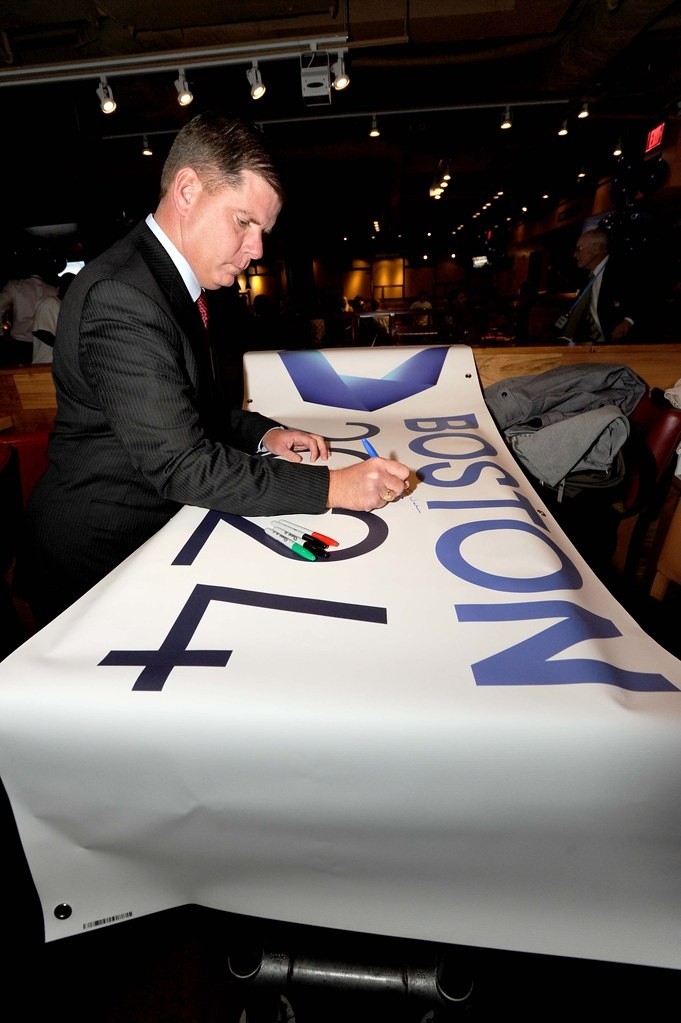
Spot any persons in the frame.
[570,232,659,345]
[411,289,433,333]
[452,289,483,337]
[2,118,409,658]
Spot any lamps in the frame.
[1,32,353,115]
[101,96,593,155]
[429,124,665,201]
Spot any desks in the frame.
[2,386,671,822]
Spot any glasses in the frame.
[575,245,590,251]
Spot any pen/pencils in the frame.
[264,527,317,561]
[270,520,326,548]
[362,438,405,500]
[269,524,330,558]
[279,519,339,548]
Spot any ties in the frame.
[195,292,207,333]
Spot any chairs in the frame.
[516,387,681,616]
